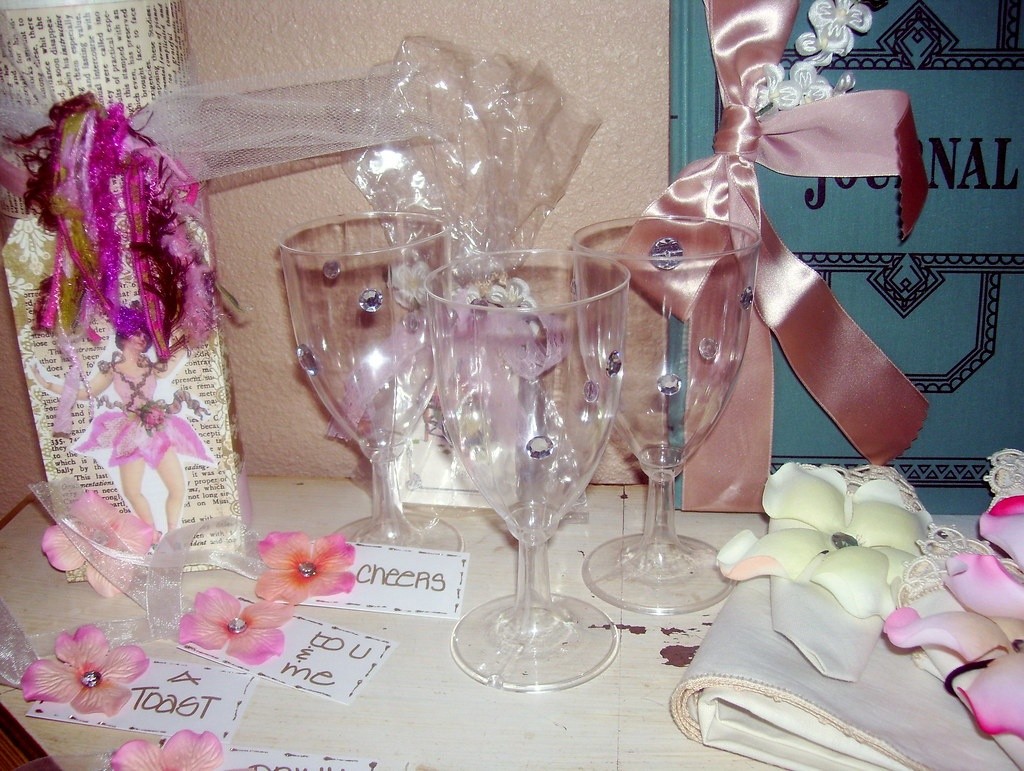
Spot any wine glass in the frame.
[279,210,463,552]
[571,216,762,616]
[423,248,631,693]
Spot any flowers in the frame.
[20,405,1023,770]
